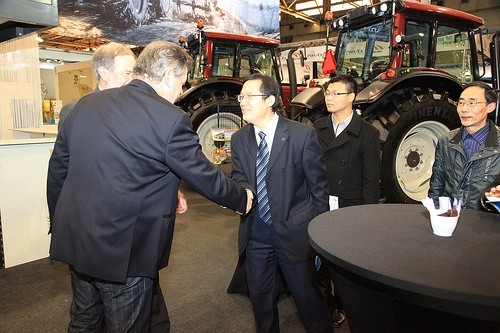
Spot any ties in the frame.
[256,131,272,226]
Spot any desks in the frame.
[8,124,60,135]
[308,204,500,333]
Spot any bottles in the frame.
[43,99,62,125]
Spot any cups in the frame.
[429,209,460,237]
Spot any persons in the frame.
[429,82,500,211]
[47,41,253,333]
[231,75,330,333]
[313,76,380,208]
[58,41,188,333]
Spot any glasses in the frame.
[456,101,488,107]
[237,94,269,102]
[324,92,352,97]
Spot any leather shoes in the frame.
[333,309,346,327]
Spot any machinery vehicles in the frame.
[291,0,500,203]
[175,20,310,177]
